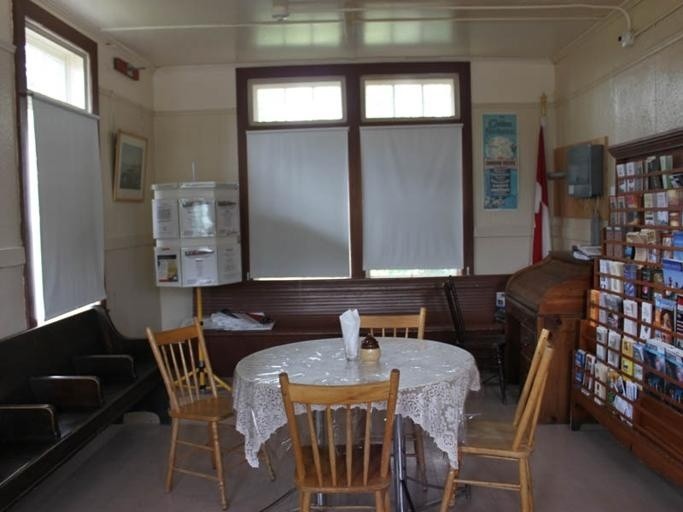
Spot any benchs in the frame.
[195,276,512,379]
[0,305,196,511]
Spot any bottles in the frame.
[360,335,379,364]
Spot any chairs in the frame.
[146,317,275,510]
[439,324,556,511]
[278,369,400,512]
[359,307,428,492]
[442,275,509,405]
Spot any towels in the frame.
[339,308,361,357]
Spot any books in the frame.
[574,155,683,428]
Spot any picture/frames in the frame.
[113,128,148,202]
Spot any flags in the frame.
[530,92,552,266]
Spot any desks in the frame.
[231,336,482,511]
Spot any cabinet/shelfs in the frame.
[568,125,682,489]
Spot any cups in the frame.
[342,341,359,360]
